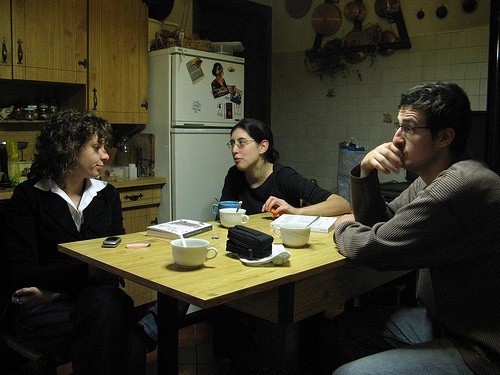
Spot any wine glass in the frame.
[18,141,31,161]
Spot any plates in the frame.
[210,42,244,51]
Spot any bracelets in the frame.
[16,296,21,306]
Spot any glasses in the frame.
[394,121,448,138]
[227,139,264,149]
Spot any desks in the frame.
[58,209,363,375]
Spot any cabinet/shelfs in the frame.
[119,185,163,304]
[0,0,150,124]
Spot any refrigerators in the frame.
[147,49,246,222]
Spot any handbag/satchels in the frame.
[225,225,273,259]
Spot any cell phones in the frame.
[102,237,121,247]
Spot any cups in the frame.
[219,207,249,228]
[274,224,311,247]
[212,200,242,222]
[171,238,218,268]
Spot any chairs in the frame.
[0,198,74,375]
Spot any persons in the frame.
[0,112,149,375]
[332,80,500,375]
[139,118,352,353]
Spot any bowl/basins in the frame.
[209,45,234,55]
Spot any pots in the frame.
[311,0,398,61]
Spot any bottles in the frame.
[0,141,21,186]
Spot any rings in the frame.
[27,288,30,293]
